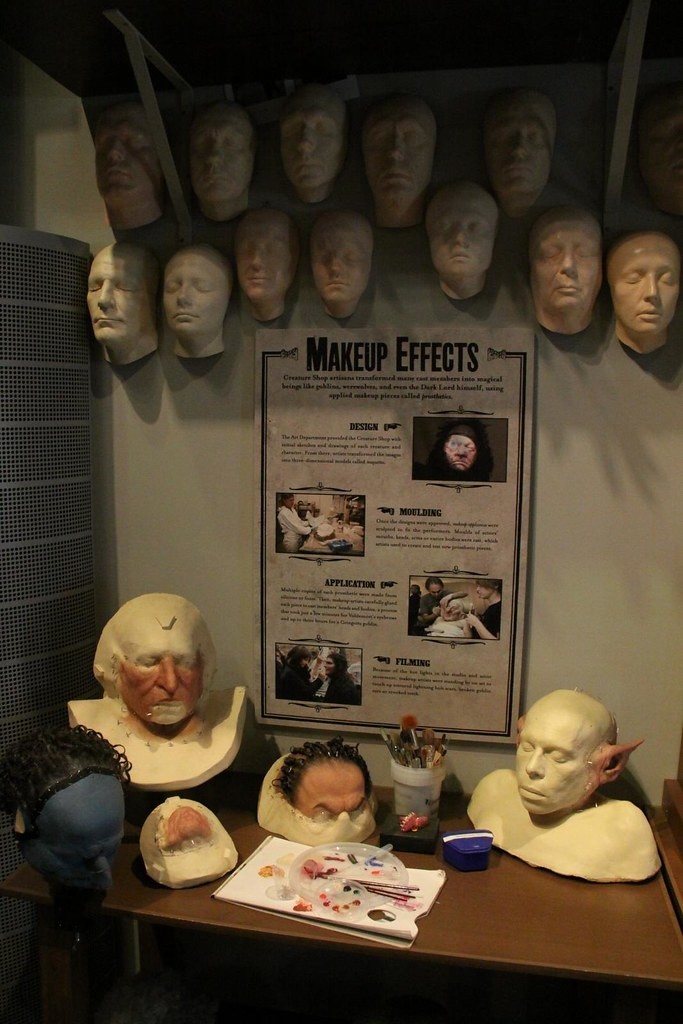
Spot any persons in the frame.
[426,180,498,299]
[94,101,165,231]
[321,652,361,705]
[362,92,437,228]
[278,82,348,204]
[636,81,683,217]
[188,101,257,222]
[278,494,312,553]
[163,243,232,358]
[606,230,680,354]
[467,689,663,883]
[307,208,373,319]
[483,88,556,219]
[409,577,501,640]
[85,240,161,365]
[0,725,132,905]
[278,645,325,700]
[235,208,300,322]
[528,205,603,334]
[427,418,493,481]
[67,592,246,792]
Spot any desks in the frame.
[0,771,683,1024]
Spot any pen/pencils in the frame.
[380,729,396,760]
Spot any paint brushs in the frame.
[319,874,420,901]
